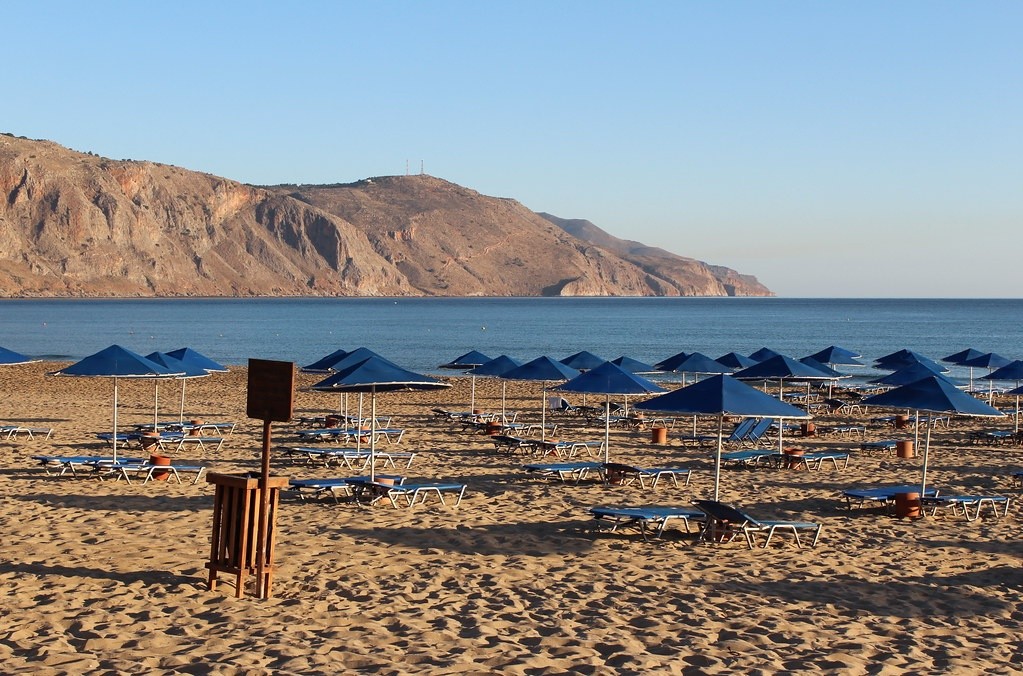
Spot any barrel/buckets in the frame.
[784,448,804,470]
[895,414,909,428]
[487,422,501,435]
[608,463,622,483]
[325,415,338,428]
[634,415,644,429]
[723,417,731,422]
[375,478,394,504]
[715,518,733,538]
[357,459,365,465]
[801,423,815,438]
[545,439,559,456]
[834,410,839,414]
[142,432,160,452]
[1018,424,1023,434]
[894,493,920,517]
[652,419,667,443]
[191,419,204,436]
[149,455,171,481]
[355,426,371,443]
[896,441,913,458]
[473,409,484,422]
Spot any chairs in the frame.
[148,435,227,451]
[287,383,1023,562]
[85,458,206,484]
[32,455,147,481]
[175,421,238,440]
[0,427,55,442]
[96,433,183,448]
[130,422,197,438]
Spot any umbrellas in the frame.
[630,373,813,540]
[142,351,212,434]
[44,344,186,464]
[163,347,230,425]
[300,345,1022,541]
[0,345,43,366]
